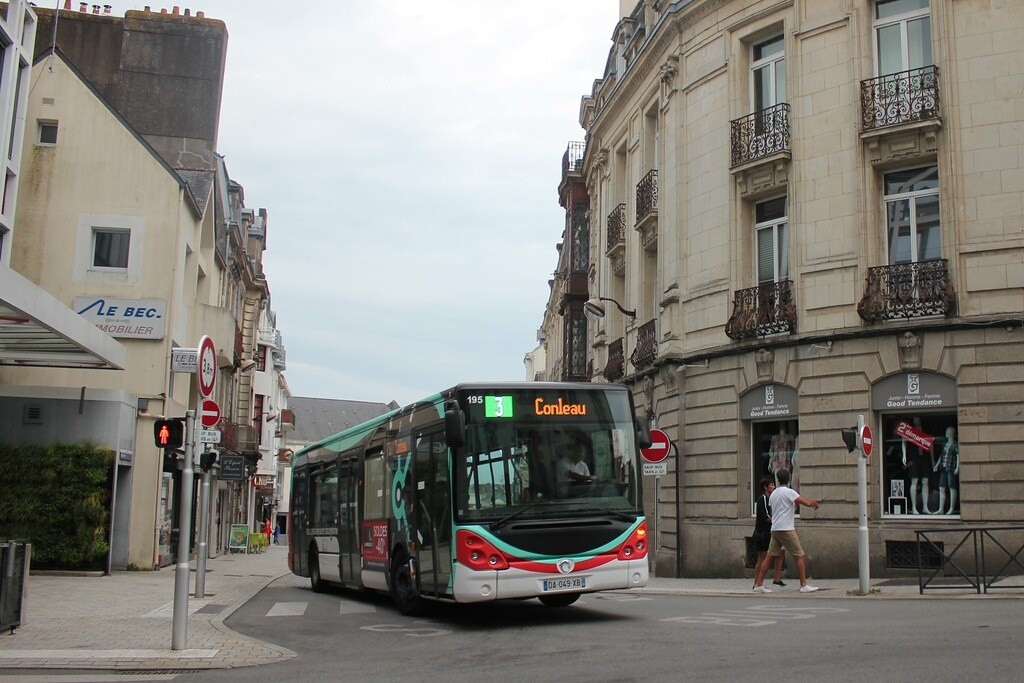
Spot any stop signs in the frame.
[859,425,873,457]
[640,430,671,463]
[202,401,221,427]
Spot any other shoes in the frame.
[773,580,787,587]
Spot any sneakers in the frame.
[799,584,819,593]
[752,584,772,593]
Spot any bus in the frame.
[287,383,653,616]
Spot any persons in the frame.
[767,424,795,489]
[753,469,819,593]
[932,427,959,515]
[553,442,599,498]
[261,517,272,546]
[895,482,902,496]
[272,521,280,545]
[752,476,789,589]
[790,432,800,514]
[901,415,935,515]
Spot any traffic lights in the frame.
[842,429,856,453]
[154,420,184,449]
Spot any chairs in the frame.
[249,533,267,554]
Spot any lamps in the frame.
[676,359,709,372]
[252,412,276,422]
[806,341,832,354]
[219,358,256,373]
[583,297,636,320]
